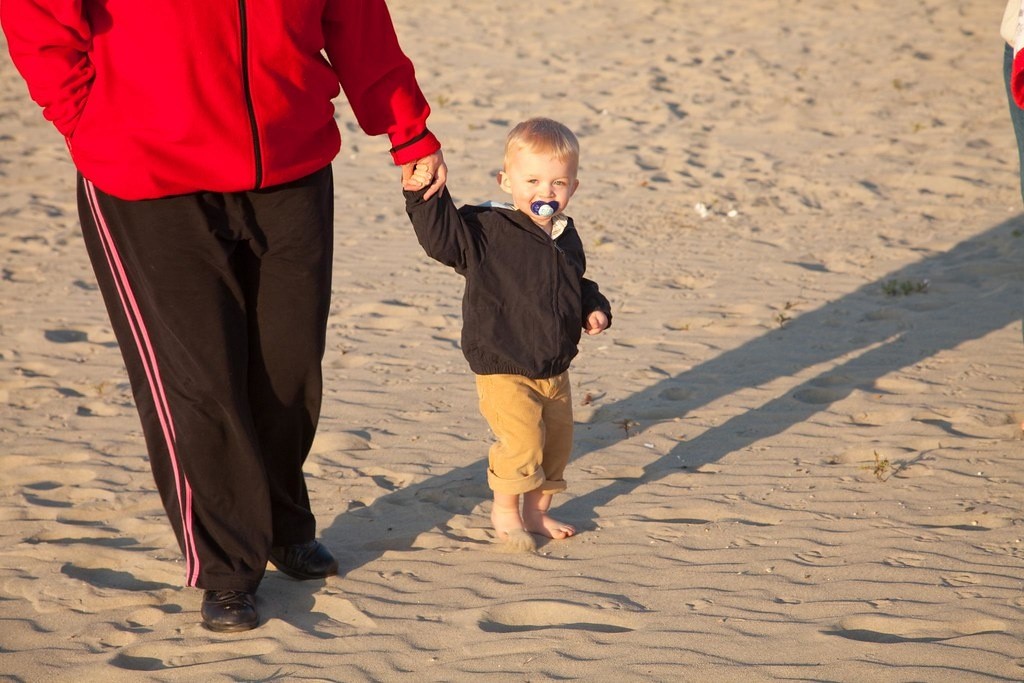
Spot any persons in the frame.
[0,0,449,631]
[1002,1,1024,198]
[402,117,612,551]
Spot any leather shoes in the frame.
[268,538,338,581]
[201,589,260,633]
